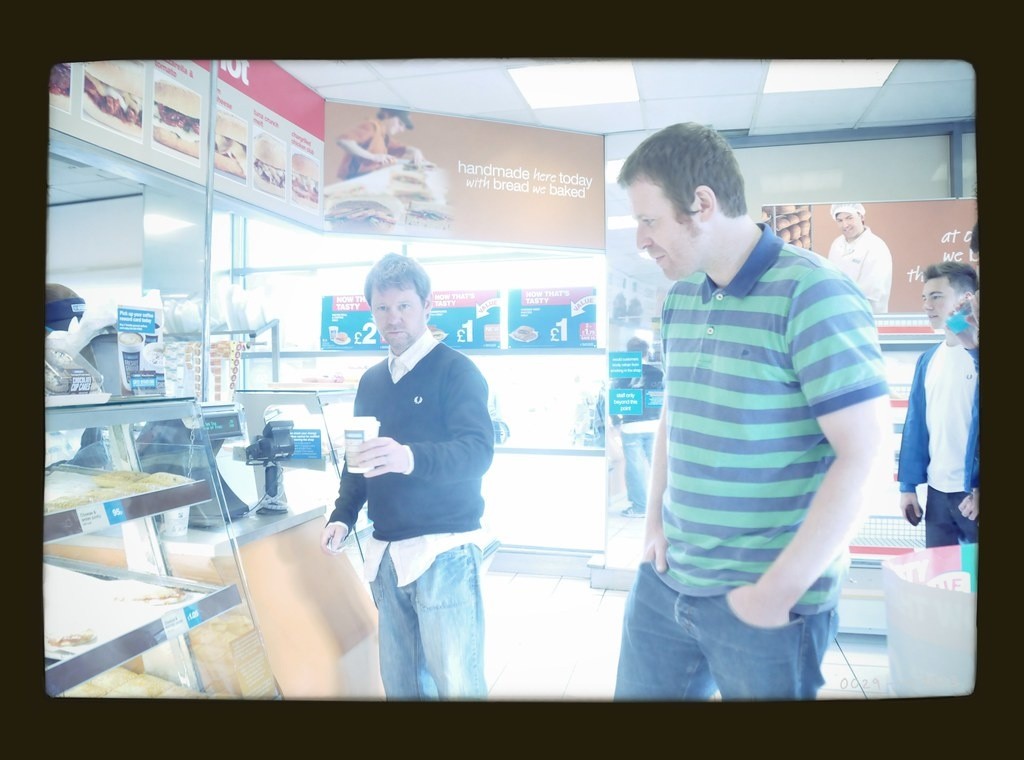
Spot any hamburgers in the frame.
[49,61,320,207]
[323,169,431,233]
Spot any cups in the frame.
[329,326,338,341]
[118,332,145,390]
[343,416,381,473]
[164,507,190,538]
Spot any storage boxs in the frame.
[426,289,501,348]
[320,295,390,349]
[507,286,596,348]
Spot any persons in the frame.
[899,263,982,548]
[822,203,893,319]
[333,108,430,177]
[611,118,887,702]
[948,220,980,375]
[609,334,664,518]
[319,252,496,700]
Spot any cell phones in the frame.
[905,503,923,525]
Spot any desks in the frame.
[43,502,390,699]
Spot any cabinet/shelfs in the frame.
[43,392,292,699]
[232,250,609,457]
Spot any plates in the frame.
[334,337,351,345]
[510,331,539,342]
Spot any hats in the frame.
[381,108,413,129]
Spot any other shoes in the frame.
[621,505,645,518]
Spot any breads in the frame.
[45,469,278,698]
[761,205,811,249]
